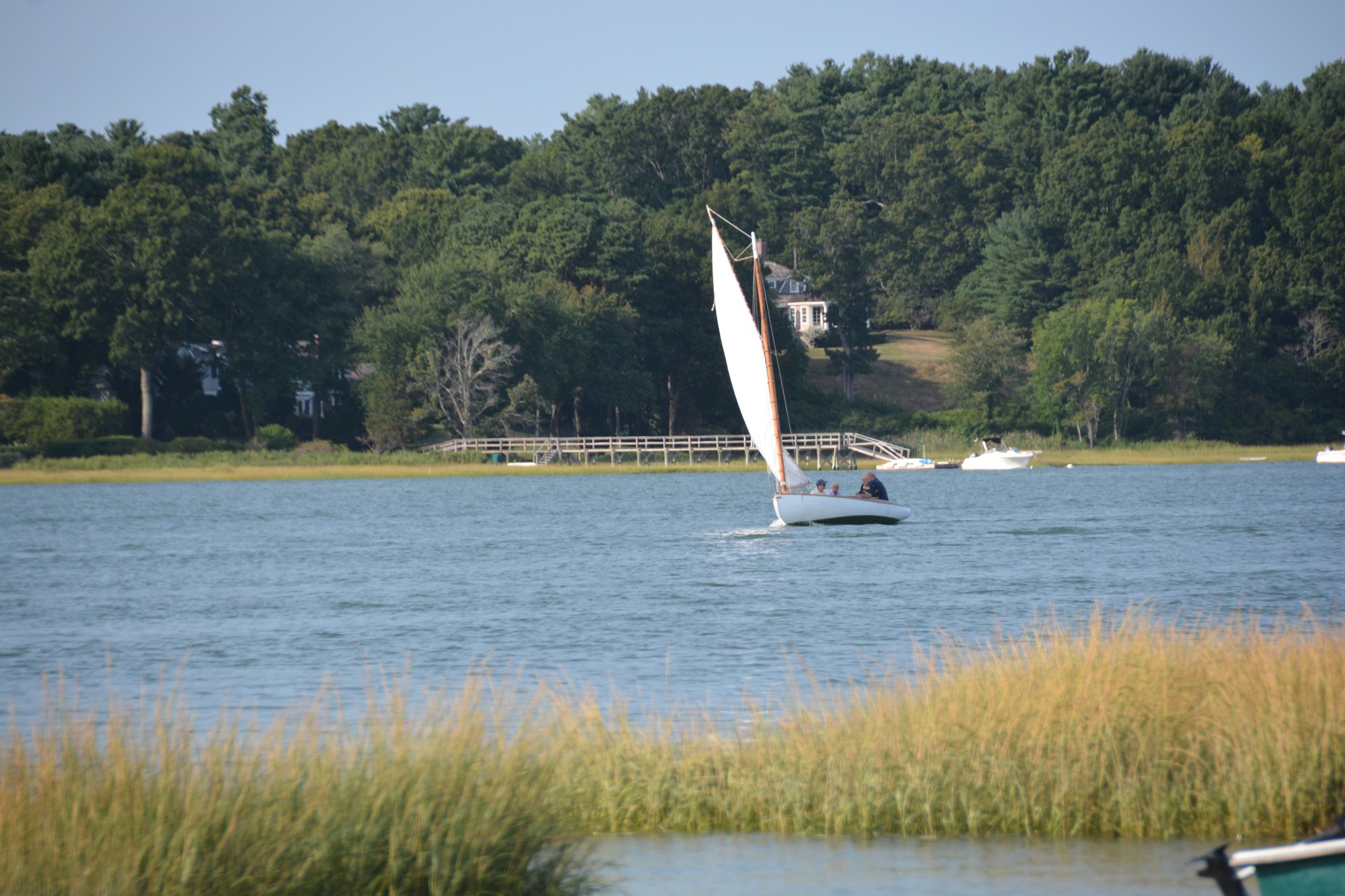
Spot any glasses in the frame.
[820,483,825,486]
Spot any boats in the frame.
[1188,825,1345,895]
[876,444,936,470]
[935,457,960,469]
[961,437,1043,471]
[1316,446,1345,463]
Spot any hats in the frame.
[816,479,828,485]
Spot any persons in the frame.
[828,482,842,496]
[810,479,828,495]
[856,471,888,501]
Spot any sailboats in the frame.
[706,203,910,527]
